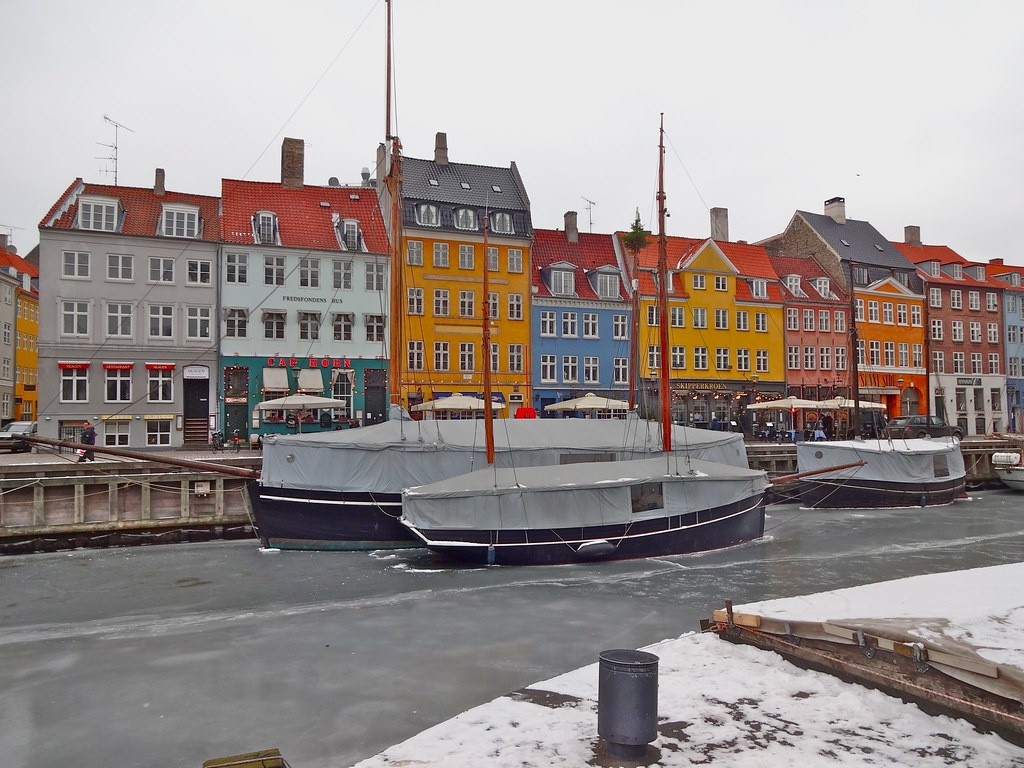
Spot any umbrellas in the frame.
[258,394,347,435]
[543,392,639,419]
[746,395,840,442]
[817,396,887,441]
[411,395,506,419]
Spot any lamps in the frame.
[649,366,661,384]
[896,375,904,390]
[742,372,759,385]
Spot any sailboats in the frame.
[796,255,969,509]
[245,0,775,568]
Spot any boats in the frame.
[992,452,1024,492]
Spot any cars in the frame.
[0,421,37,454]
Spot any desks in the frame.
[815,430,826,439]
[759,431,769,437]
[787,431,796,441]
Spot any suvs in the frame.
[885,414,964,441]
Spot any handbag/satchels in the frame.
[76,449,86,457]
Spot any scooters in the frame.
[208,427,225,454]
[229,428,243,453]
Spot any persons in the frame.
[85,423,98,461]
[821,412,834,440]
[77,421,92,462]
[709,417,720,431]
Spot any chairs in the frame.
[249,434,261,450]
[753,428,836,443]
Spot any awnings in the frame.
[433,392,506,405]
